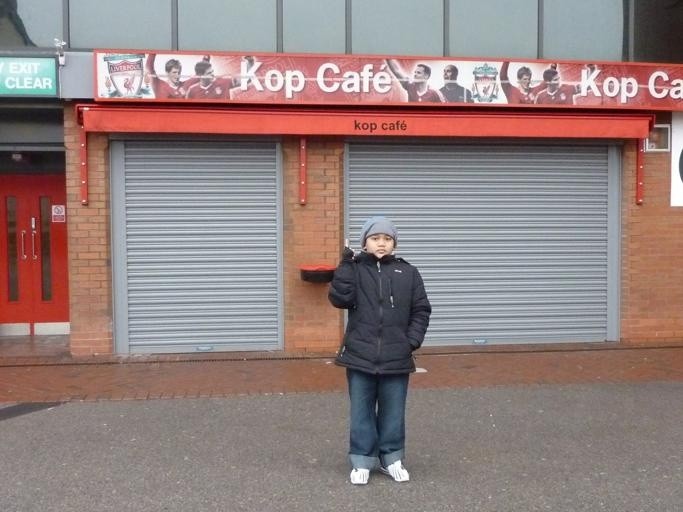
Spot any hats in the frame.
[361,218,398,251]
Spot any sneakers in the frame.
[349,466,370,483]
[381,458,410,482]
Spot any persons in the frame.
[185,56,256,100]
[384,59,440,103]
[144,53,210,99]
[437,65,471,103]
[326,217,431,486]
[498,61,557,105]
[535,64,596,105]
